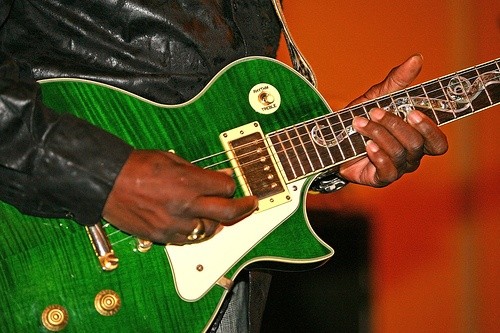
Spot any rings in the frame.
[185,217,208,242]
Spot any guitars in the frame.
[0,53,500,333]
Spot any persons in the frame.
[2,1,451,333]
